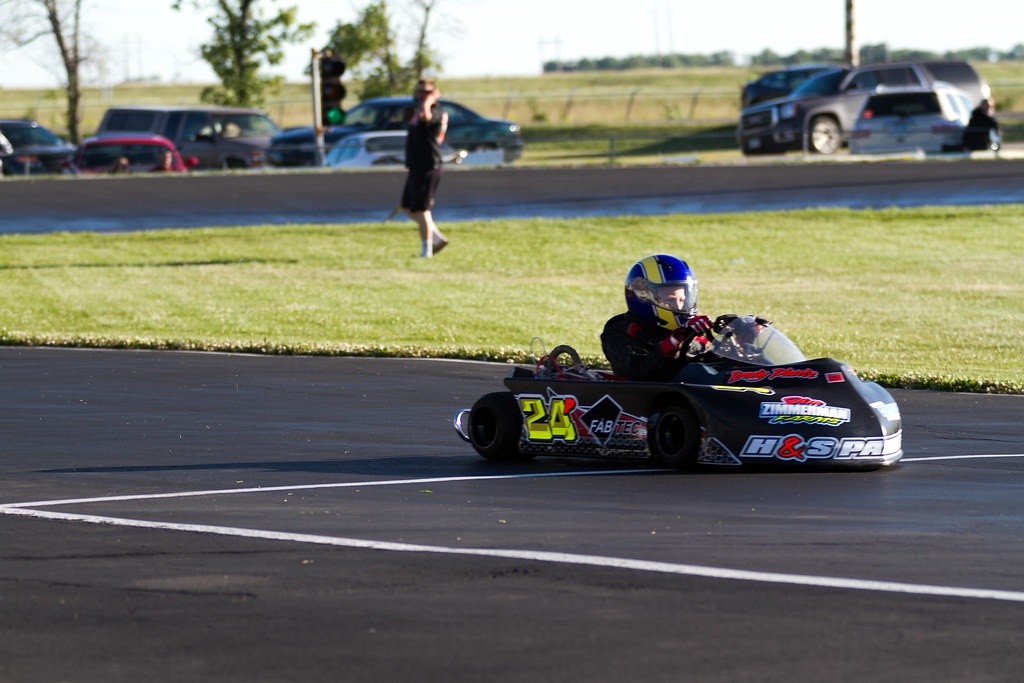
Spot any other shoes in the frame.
[433,239,449,254]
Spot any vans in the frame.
[95,107,283,173]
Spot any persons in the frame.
[598,256,763,382]
[961,98,999,153]
[153,149,179,171]
[401,79,449,258]
[108,157,133,174]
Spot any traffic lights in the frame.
[318,58,347,126]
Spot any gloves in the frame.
[750,322,765,336]
[673,315,713,342]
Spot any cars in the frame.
[735,63,832,107]
[847,80,1002,159]
[327,134,462,168]
[0,118,83,175]
[270,96,524,168]
[56,132,198,174]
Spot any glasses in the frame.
[416,89,432,95]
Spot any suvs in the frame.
[735,58,999,157]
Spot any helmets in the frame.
[625,254,698,331]
